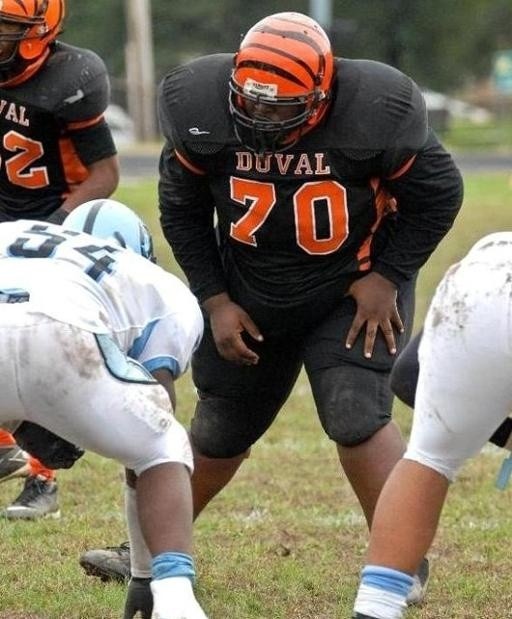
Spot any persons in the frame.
[0,0,118,519]
[1,197,211,619]
[82,12,464,606]
[351,232,512,619]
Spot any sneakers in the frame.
[404,558,429,609]
[0,445,32,482]
[2,474,61,522]
[78,542,132,584]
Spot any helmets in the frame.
[227,11,334,146]
[62,198,154,264]
[1,0,65,88]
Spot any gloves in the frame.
[123,570,153,619]
